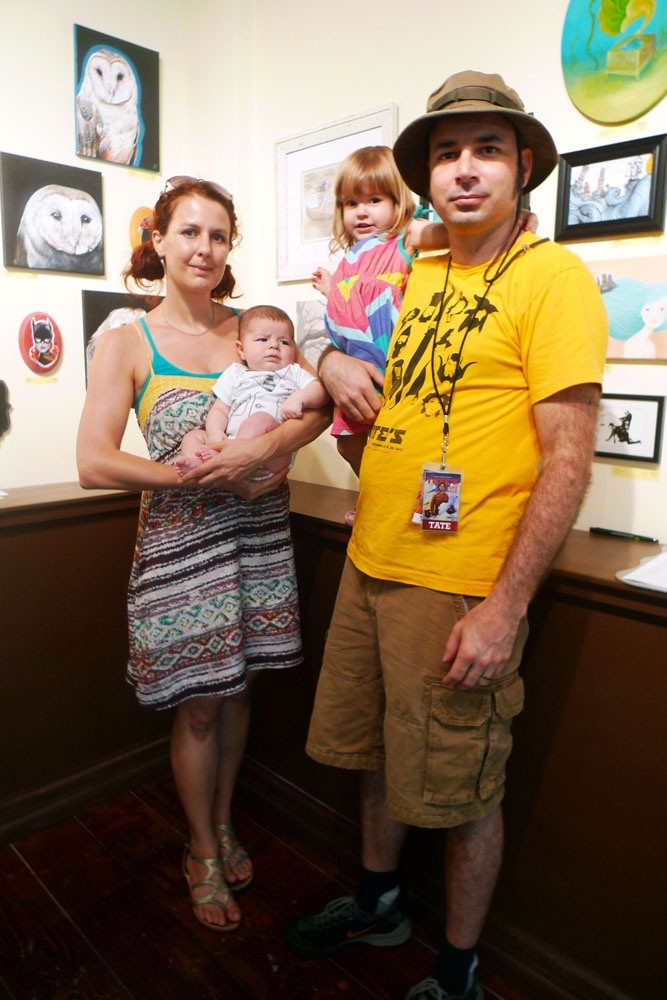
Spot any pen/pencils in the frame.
[588,527,660,544]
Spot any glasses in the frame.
[162,175,235,203]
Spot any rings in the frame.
[213,481,218,487]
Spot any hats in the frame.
[393,70,558,198]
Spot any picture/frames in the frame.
[273,103,399,284]
[593,393,663,462]
[554,132,667,242]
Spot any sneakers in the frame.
[283,892,411,955]
[404,934,485,999]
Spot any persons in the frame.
[288,71,610,1000]
[173,305,329,483]
[70,174,333,934]
[311,145,545,528]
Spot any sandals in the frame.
[214,819,254,892]
[182,838,240,930]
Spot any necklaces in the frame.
[160,296,215,337]
